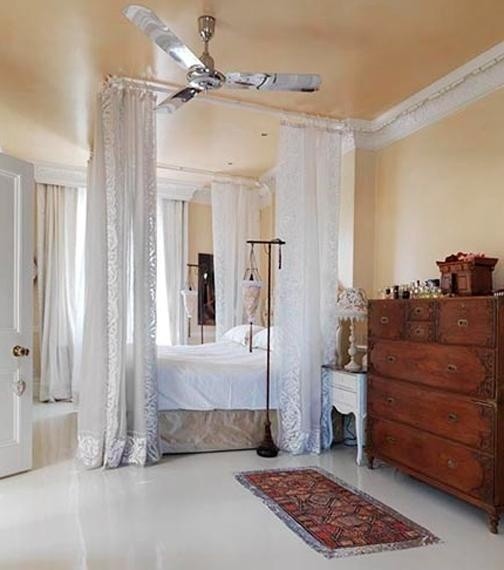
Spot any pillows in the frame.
[225,324,274,352]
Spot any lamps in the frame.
[181,260,211,346]
[241,240,287,457]
[330,288,369,372]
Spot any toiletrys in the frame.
[383,280,441,298]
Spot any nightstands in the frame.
[328,365,368,466]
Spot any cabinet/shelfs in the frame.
[364,296,504,534]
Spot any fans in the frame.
[121,5,322,115]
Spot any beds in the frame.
[126,337,279,456]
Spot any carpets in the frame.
[232,464,443,561]
